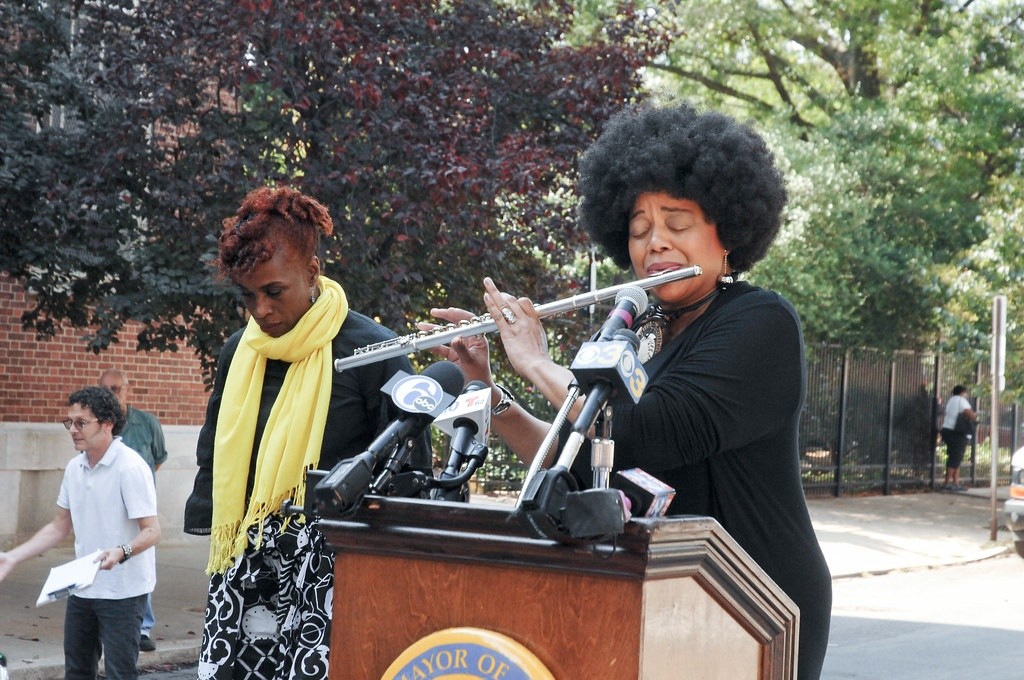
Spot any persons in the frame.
[182,186,435,680]
[424,100,832,680]
[99,368,167,651]
[0,386,161,680]
[940,385,978,492]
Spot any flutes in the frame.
[331,262,707,373]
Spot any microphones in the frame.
[442,381,489,482]
[364,360,465,462]
[557,328,641,473]
[567,284,649,398]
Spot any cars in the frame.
[1003,446,1024,560]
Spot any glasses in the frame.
[62,418,101,430]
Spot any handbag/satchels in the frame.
[194,499,339,680]
[954,412,977,436]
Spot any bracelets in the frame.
[491,383,516,417]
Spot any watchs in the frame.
[115,544,133,564]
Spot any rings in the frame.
[502,307,516,325]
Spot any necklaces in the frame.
[636,284,724,365]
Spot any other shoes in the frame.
[940,483,969,492]
[139,633,156,652]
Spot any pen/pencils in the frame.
[48,584,75,595]
